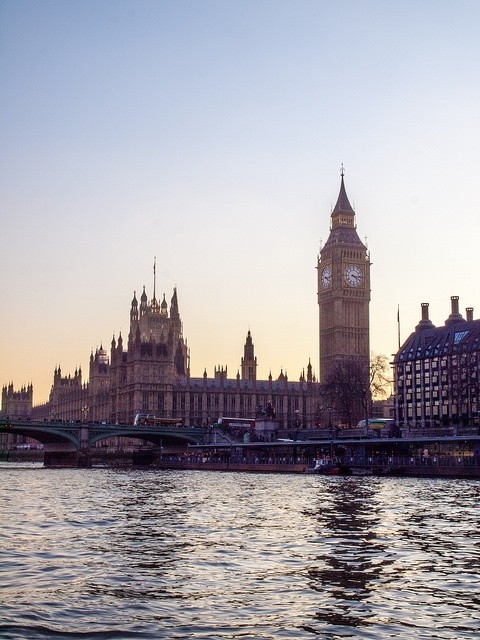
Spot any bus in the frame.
[134,414,182,429]
[357,418,394,430]
[218,416,255,430]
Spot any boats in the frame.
[306,458,339,475]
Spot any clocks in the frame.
[343,263,364,288]
[320,266,332,289]
